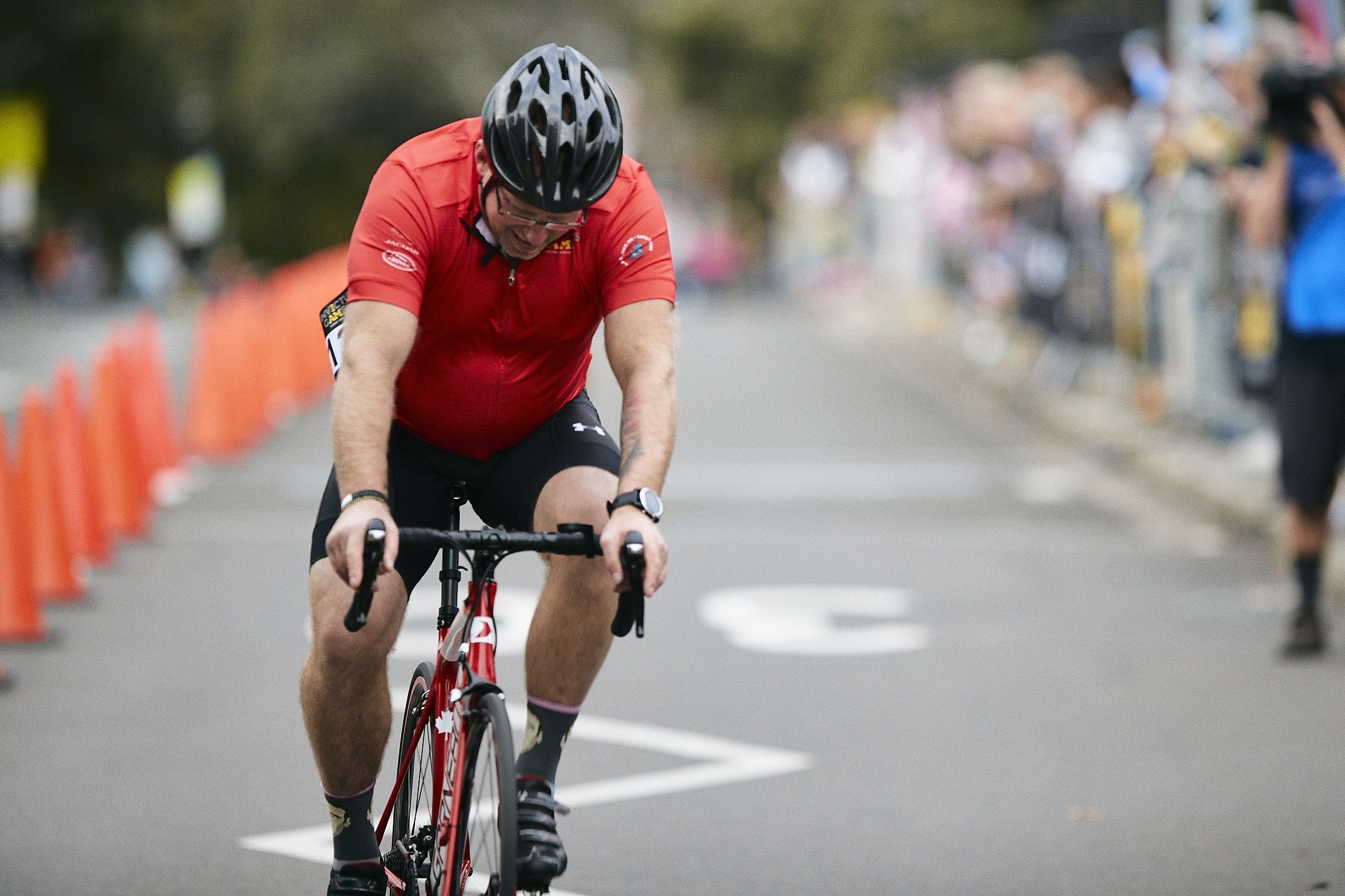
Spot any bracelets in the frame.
[340,490,391,509]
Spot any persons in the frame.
[301,44,676,896]
[751,1,1345,662]
[19,222,189,330]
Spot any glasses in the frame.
[493,181,588,232]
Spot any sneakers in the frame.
[497,774,567,882]
[326,848,388,896]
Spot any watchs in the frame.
[606,486,663,522]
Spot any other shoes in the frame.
[1283,608,1324,657]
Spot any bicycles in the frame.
[338,483,644,896]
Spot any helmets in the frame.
[482,43,623,209]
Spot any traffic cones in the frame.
[2,247,358,649]
